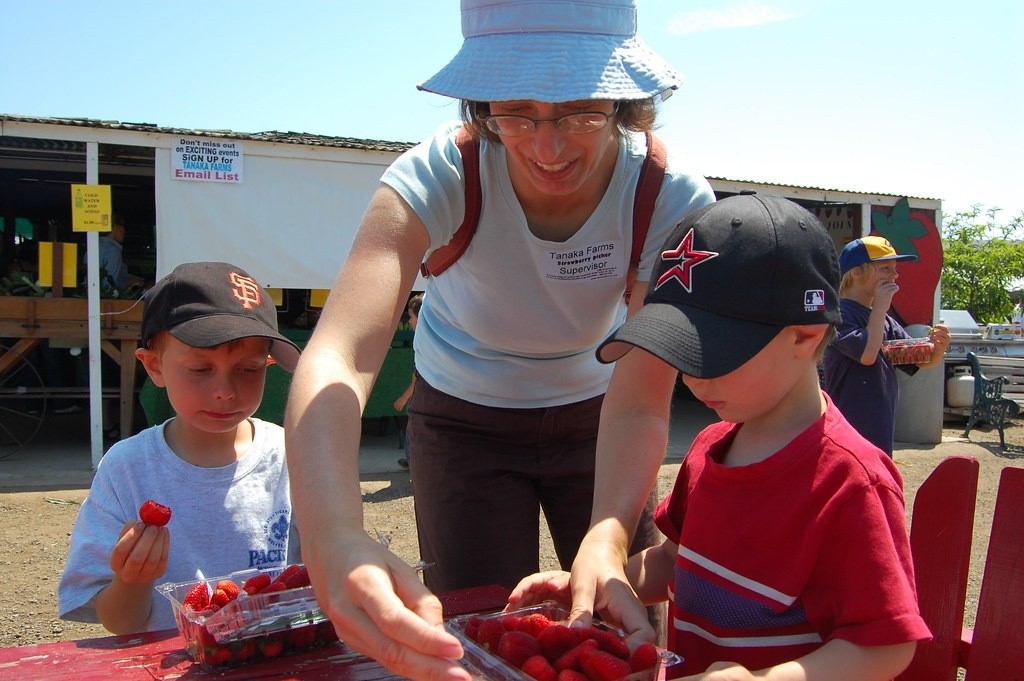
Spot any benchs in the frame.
[960,352,1020,448]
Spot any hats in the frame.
[417,0,682,102]
[142,263,302,374]
[596,187,841,380]
[840,237,918,275]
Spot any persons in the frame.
[78,214,156,443]
[501,190,933,681]
[395,293,422,467]
[285,0,716,681]
[820,235,951,460]
[58,261,304,635]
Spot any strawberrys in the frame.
[463,613,657,681]
[882,341,932,365]
[177,563,340,666]
[139,500,172,526]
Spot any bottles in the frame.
[1012,313,1024,338]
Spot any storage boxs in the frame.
[153,567,346,668]
[882,337,932,366]
[444,600,684,681]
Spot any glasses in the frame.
[474,101,622,137]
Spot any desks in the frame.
[0,584,511,681]
